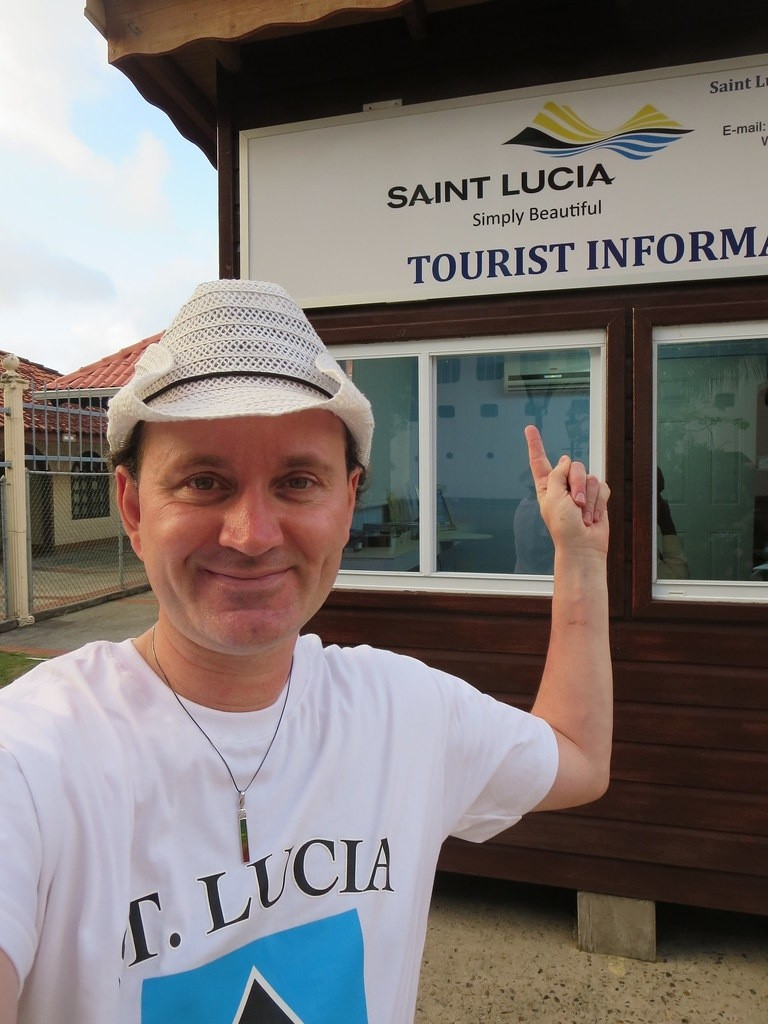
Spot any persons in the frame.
[0,278,615,1024]
[512,452,559,573]
[656,466,688,580]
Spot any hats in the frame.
[105,280,373,468]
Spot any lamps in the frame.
[63,434,77,442]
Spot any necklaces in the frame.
[153,619,295,863]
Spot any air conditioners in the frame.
[503,359,590,393]
